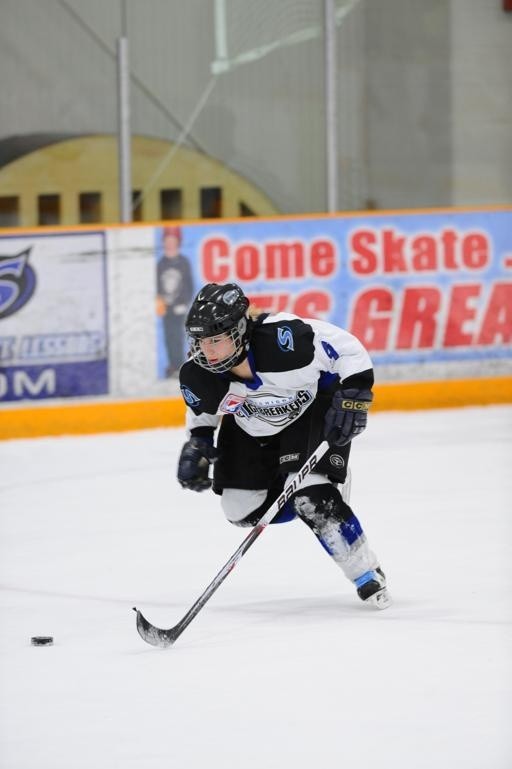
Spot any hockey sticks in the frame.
[136,427,341,648]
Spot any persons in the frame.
[155,227,196,380]
[178,282,388,602]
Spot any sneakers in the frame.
[356,569,386,600]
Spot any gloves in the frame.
[177,438,219,490]
[322,390,372,446]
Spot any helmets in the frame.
[185,284,252,375]
